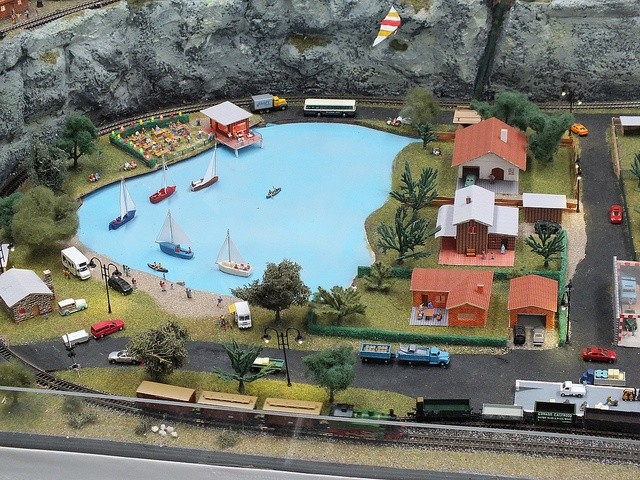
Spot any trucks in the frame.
[91,319,124,340]
[61,246,91,280]
[250,93,288,113]
[561,381,585,398]
[61,329,88,346]
[249,357,285,375]
[579,369,626,387]
[359,343,450,369]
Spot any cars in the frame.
[464,174,477,187]
[533,328,545,346]
[58,298,88,315]
[620,276,636,304]
[513,325,525,345]
[107,349,141,365]
[610,205,623,224]
[570,123,589,136]
[623,310,637,331]
[534,218,562,234]
[582,348,616,364]
[108,276,133,296]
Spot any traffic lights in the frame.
[65,346,76,358]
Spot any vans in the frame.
[228,302,252,329]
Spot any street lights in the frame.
[0,240,14,273]
[263,327,303,387]
[561,90,582,112]
[88,257,122,313]
[561,282,572,344]
[575,177,583,213]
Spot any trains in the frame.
[135,380,407,442]
[407,397,640,437]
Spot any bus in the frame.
[303,98,356,117]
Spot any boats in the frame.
[147,263,169,273]
[266,188,281,198]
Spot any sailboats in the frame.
[215,229,252,277]
[108,176,137,230]
[191,141,218,192]
[149,155,176,203]
[156,209,194,259]
